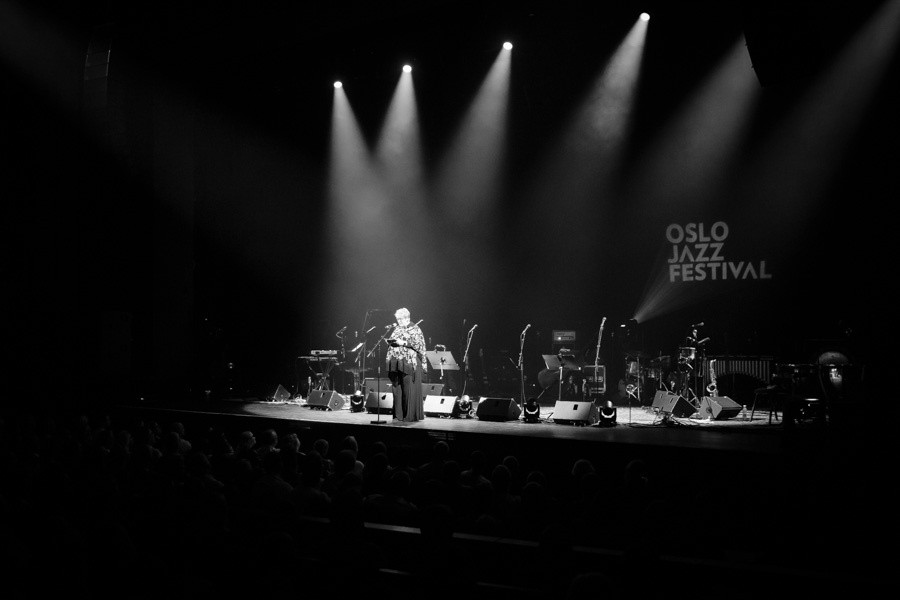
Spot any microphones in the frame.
[469,324,478,332]
[600,316,606,327]
[692,321,704,328]
[337,326,347,335]
[522,324,531,334]
[385,323,397,328]
[366,326,377,333]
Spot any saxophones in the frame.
[706,359,720,398]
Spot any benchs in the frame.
[0,435,900,600]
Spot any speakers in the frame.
[421,382,449,401]
[553,400,599,427]
[651,390,697,418]
[423,395,460,419]
[268,384,291,402]
[364,392,393,415]
[698,395,743,421]
[307,390,345,410]
[476,396,521,422]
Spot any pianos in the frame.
[298,349,343,391]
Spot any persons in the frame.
[386,307,429,425]
[0,399,735,565]
